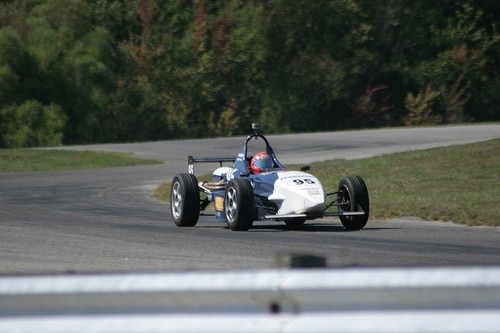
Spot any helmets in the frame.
[251,151,274,174]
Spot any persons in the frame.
[250,152,275,174]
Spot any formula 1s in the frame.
[169,123,370,230]
